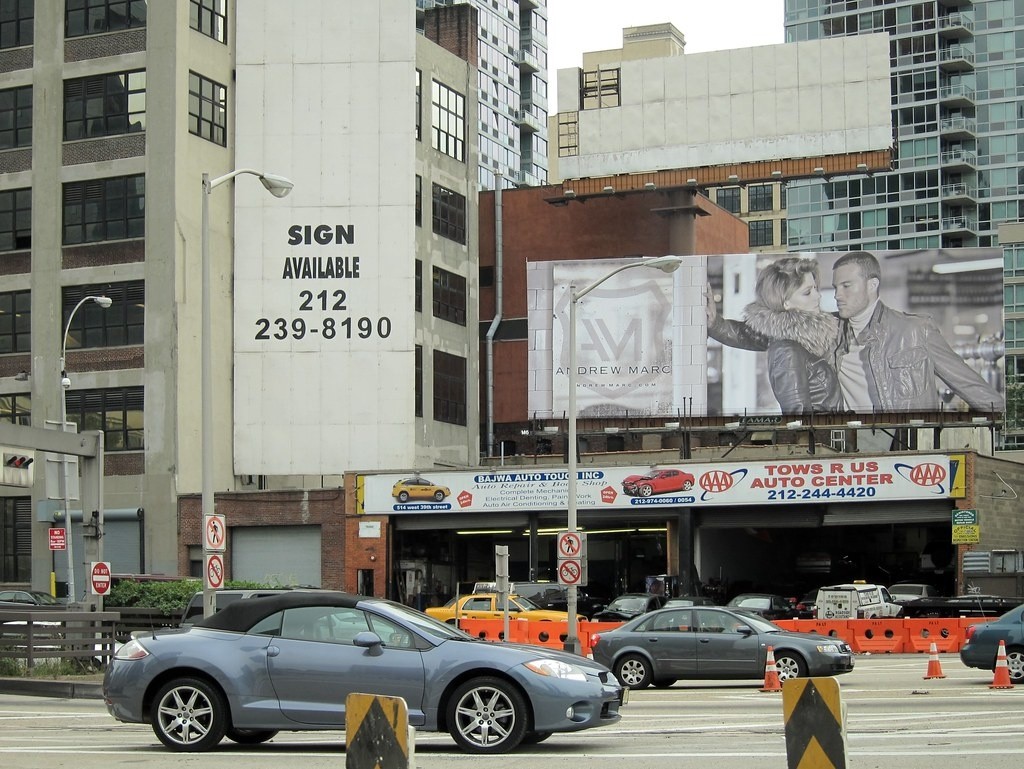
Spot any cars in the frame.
[885,582,936,602]
[593,593,667,625]
[657,596,717,624]
[424,594,587,629]
[321,612,404,642]
[960,601,1023,684]
[592,603,856,692]
[728,593,814,625]
[621,468,693,499]
[389,477,450,503]
[0,590,67,625]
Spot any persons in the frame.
[708,251,1002,417]
[742,256,846,416]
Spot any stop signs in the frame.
[91,561,112,594]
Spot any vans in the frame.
[474,582,597,620]
[814,578,901,625]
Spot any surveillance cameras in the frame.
[62,378,71,389]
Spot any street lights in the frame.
[201,166,295,621]
[565,254,682,655]
[62,296,113,605]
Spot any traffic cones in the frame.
[757,640,1014,692]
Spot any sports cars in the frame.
[101,593,630,752]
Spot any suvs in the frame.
[183,589,343,622]
